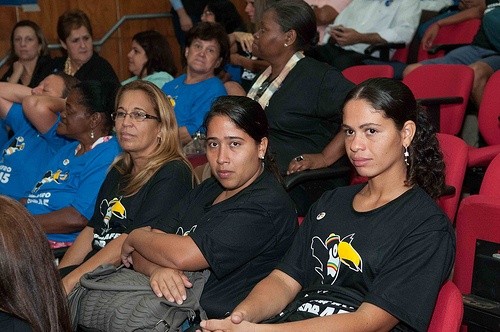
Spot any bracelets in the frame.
[435,21,441,28]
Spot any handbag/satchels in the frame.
[65,264,211,332]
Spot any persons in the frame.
[120,95,301,332]
[0,0,500,332]
[195,77,456,332]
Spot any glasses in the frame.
[111,110,160,122]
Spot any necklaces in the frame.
[256,74,280,95]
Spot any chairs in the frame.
[288,4,500,332]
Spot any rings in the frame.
[295,155,304,161]
